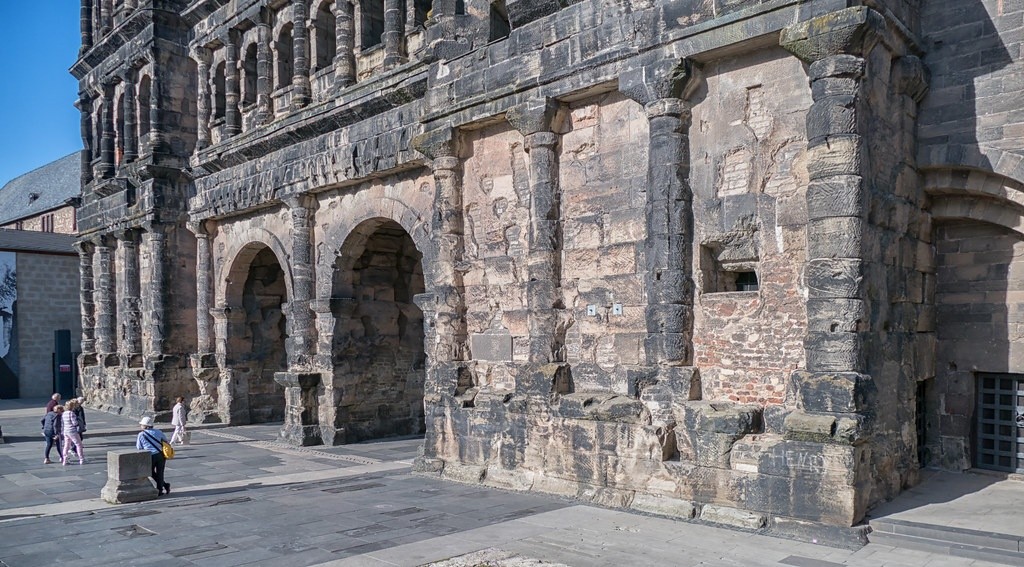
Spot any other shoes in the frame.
[44,459,52,464]
[165,482,172,495]
[59,456,69,463]
[63,461,70,466]
[80,458,89,464]
[159,488,162,496]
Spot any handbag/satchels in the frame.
[163,442,173,459]
[76,418,86,433]
[177,430,191,445]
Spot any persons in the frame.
[68,396,86,456]
[41,405,63,464]
[136,417,171,496]
[61,401,85,465]
[168,397,186,447]
[46,393,61,412]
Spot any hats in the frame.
[138,417,153,427]
